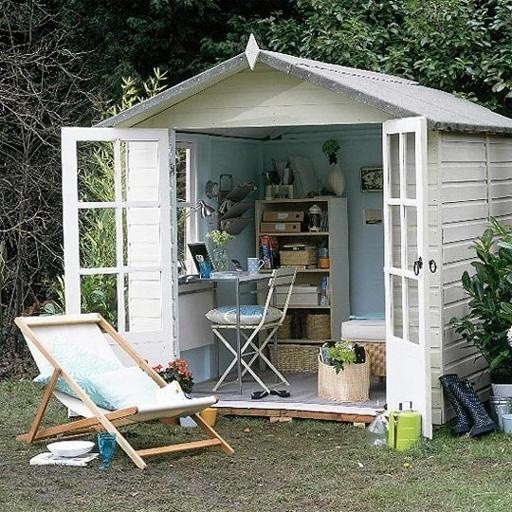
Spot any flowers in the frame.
[152,358,194,395]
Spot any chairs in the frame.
[210,264,298,395]
[11,311,235,472]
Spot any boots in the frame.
[438,374,499,438]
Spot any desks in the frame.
[197,271,281,396]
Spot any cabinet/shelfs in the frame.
[254,195,349,371]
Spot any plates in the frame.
[46,441,95,458]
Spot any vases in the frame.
[502,414,512,434]
[158,413,179,427]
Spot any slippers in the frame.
[251,390,268,399]
[270,387,290,397]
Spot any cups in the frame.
[199,260,211,278]
[247,258,265,276]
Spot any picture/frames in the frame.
[359,167,383,193]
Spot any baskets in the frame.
[267,343,320,376]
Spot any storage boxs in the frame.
[260,209,322,307]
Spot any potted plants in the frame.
[205,230,236,269]
[319,139,347,196]
[448,216,512,426]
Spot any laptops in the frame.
[186,242,249,280]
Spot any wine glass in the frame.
[96,433,118,470]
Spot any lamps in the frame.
[177,199,216,225]
